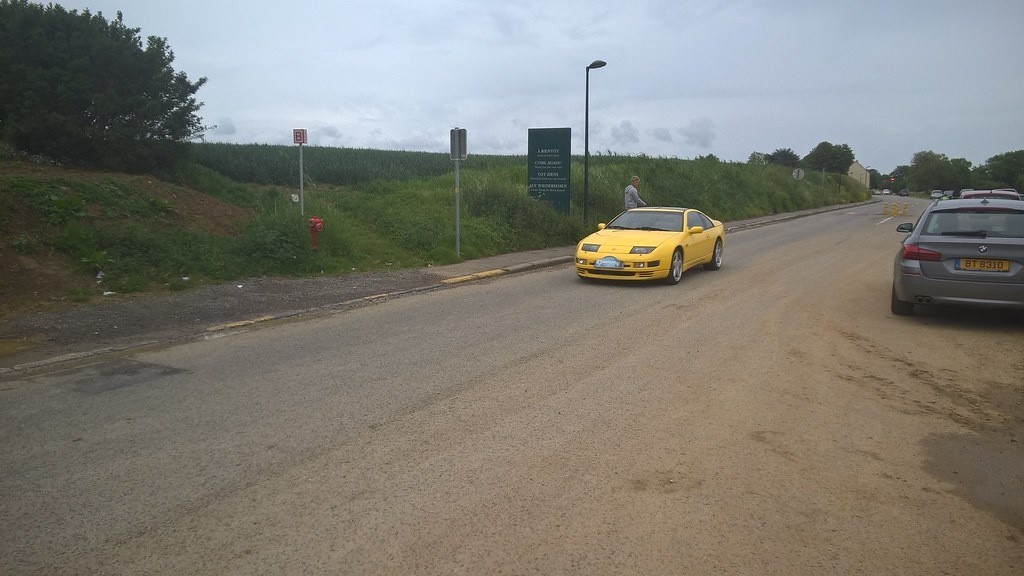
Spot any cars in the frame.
[960,189,1020,200]
[870,188,955,199]
[890,195,1024,323]
[576,206,726,286]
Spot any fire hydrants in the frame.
[308,215,325,251]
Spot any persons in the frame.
[623,176,648,210]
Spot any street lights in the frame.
[582,59,607,227]
[865,166,870,188]
